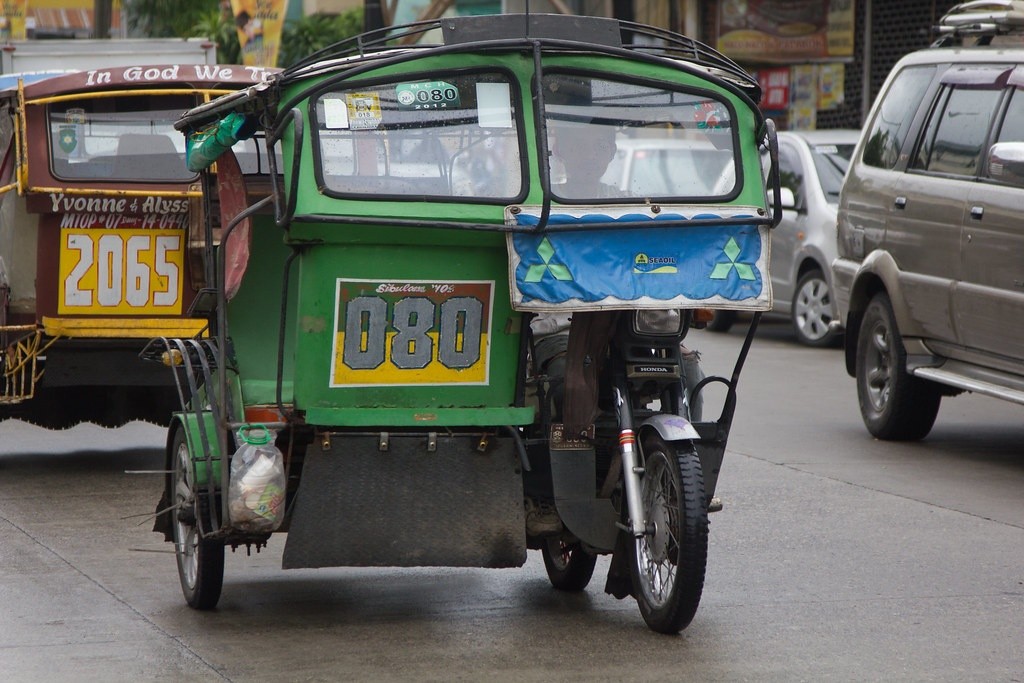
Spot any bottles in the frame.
[227,424,285,530]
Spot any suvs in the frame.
[830,1,1023,443]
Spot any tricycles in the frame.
[155,14,785,635]
[0,63,289,432]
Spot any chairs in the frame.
[115,134,183,179]
[224,213,294,425]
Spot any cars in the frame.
[703,127,864,349]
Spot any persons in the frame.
[530,110,725,514]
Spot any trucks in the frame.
[1,37,218,76]
